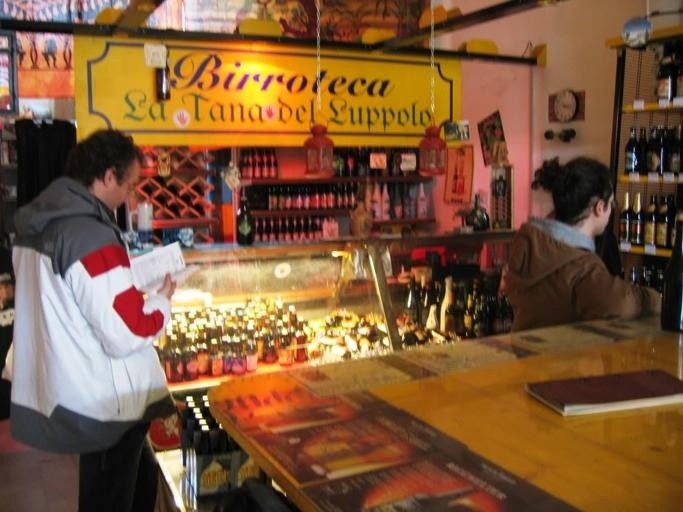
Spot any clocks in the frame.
[554,88,577,121]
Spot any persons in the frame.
[504,155,662,333]
[9,129,179,512]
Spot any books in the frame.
[526,368,683,418]
[132,241,200,294]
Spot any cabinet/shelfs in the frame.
[239,176,365,221]
[365,175,436,228]
[596,33,683,259]
[127,165,218,230]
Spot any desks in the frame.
[207,315,683,512]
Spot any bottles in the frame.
[156,50,170,99]
[470,193,490,230]
[155,262,517,388]
[615,52,683,333]
[449,148,465,195]
[181,393,239,468]
[232,146,443,247]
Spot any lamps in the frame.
[417,0,447,177]
[302,0,334,175]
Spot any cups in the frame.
[127,204,155,250]
